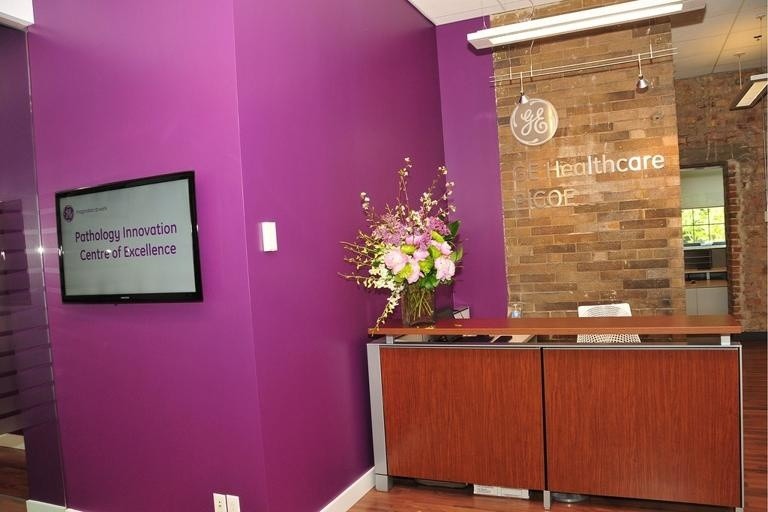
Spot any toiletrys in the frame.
[511,303,522,318]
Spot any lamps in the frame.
[729,14,768,111]
[467,0,706,50]
[489,44,679,104]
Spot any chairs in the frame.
[577,303,643,344]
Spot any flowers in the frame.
[337,157,468,336]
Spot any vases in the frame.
[402,279,435,327]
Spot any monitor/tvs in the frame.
[55,170,203,304]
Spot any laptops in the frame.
[431,306,493,342]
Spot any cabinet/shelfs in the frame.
[683,245,727,315]
